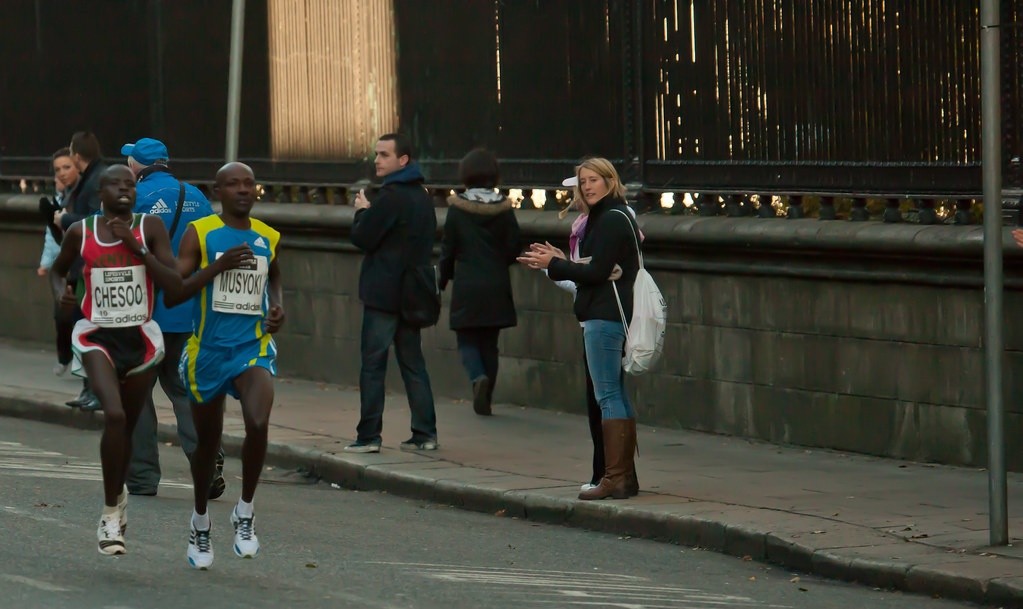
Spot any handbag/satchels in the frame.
[399,255,442,327]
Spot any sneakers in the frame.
[117,484,129,535]
[400,435,438,450]
[97,510,126,555]
[345,438,383,452]
[230,503,261,558]
[185,513,215,570]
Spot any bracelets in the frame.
[138,247,147,258]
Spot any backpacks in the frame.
[606,208,667,375]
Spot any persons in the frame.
[39,147,83,374]
[164,161,285,571]
[53,131,110,411]
[95,138,226,499]
[438,150,521,416]
[345,134,438,453]
[515,158,644,500]
[48,164,183,555]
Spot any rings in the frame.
[535,263,537,265]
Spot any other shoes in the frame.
[65,387,95,406]
[53,362,67,374]
[82,399,102,413]
[473,376,492,416]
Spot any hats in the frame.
[562,176,579,187]
[120,138,170,165]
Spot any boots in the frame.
[579,417,638,500]
